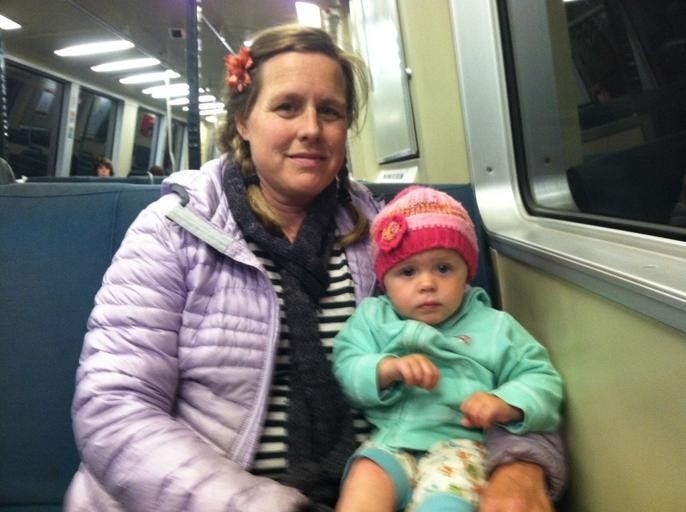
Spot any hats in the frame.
[369,186,479,291]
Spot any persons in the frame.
[92,157,114,178]
[58,22,572,511]
[589,79,612,103]
[327,186,569,511]
